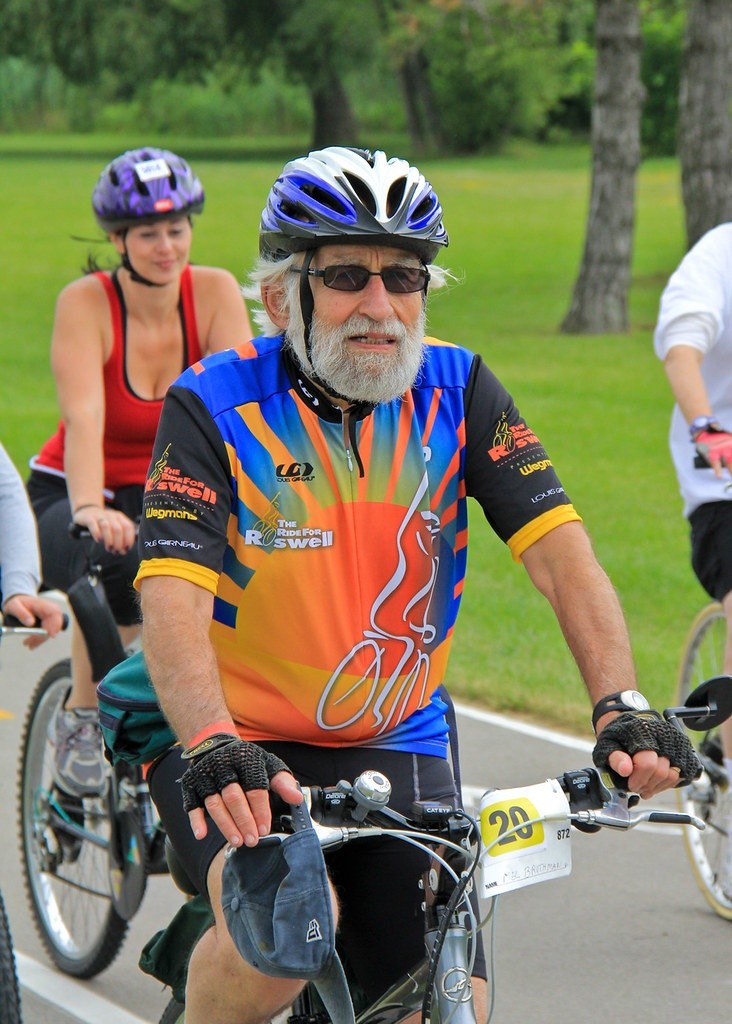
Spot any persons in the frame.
[0,438,61,650]
[653,220,732,927]
[25,146,261,799]
[132,147,705,1023]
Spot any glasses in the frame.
[289,264,431,294]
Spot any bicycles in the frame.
[672,452,732,918]
[0,602,71,1024]
[15,523,308,1020]
[155,757,713,1024]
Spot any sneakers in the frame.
[46,686,110,799]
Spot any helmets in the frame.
[259,146,450,266]
[90,147,205,234]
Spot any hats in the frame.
[220,781,358,1023]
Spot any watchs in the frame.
[689,413,718,441]
[591,689,649,732]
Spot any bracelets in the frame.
[74,504,104,513]
